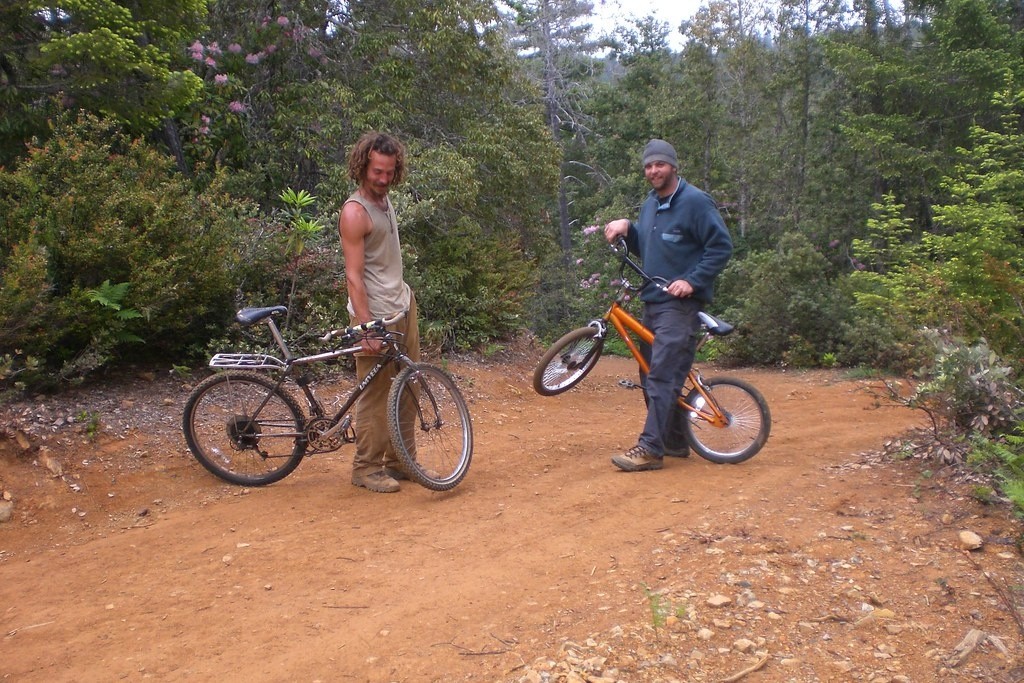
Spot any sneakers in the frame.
[611,445,663,472]
[385,462,441,483]
[664,446,690,457]
[351,470,400,493]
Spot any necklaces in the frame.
[364,186,395,234]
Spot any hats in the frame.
[643,139,678,168]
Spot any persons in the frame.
[339,130,442,494]
[605,139,733,471]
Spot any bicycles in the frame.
[180,303,475,491]
[530,226,772,465]
